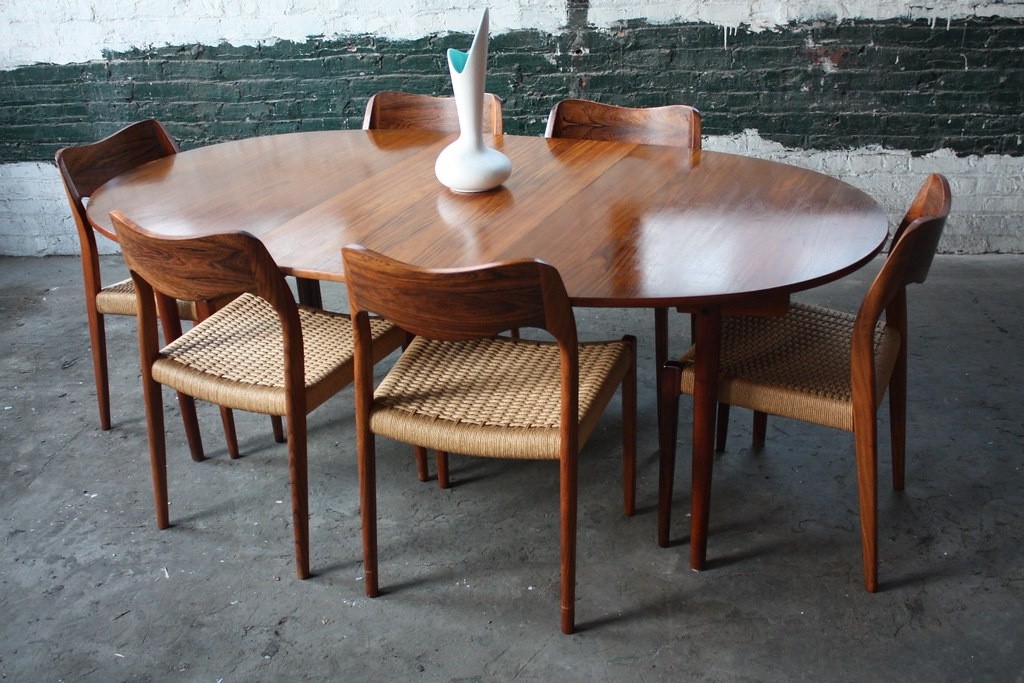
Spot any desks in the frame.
[86,129,889,548]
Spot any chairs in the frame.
[341,243,638,634]
[657,171,951,592]
[107,208,429,581]
[361,91,505,135]
[509,100,702,381]
[55,116,288,459]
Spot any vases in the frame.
[434,6,512,192]
